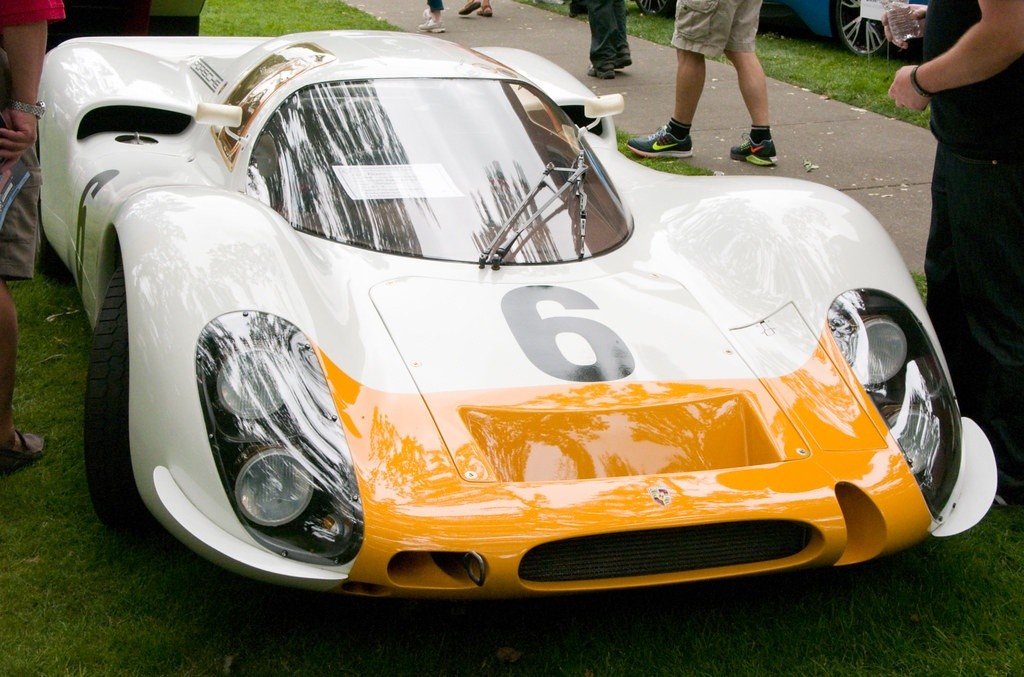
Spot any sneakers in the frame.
[588,64,615,79]
[423,9,433,21]
[419,19,446,33]
[626,123,693,158]
[730,133,777,166]
[613,52,632,69]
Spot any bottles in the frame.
[880,0,921,41]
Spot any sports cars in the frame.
[31,26,1003,600]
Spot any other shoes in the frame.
[0,429,46,475]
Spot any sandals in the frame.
[458,0,481,15]
[477,5,492,16]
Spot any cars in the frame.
[634,0,928,62]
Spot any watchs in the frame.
[6,99,47,120]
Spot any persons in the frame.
[418,0,447,33]
[626,0,779,166]
[584,0,632,80]
[459,0,492,17]
[569,0,588,17]
[0,0,66,473]
[878,0,1024,510]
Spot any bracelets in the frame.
[910,65,934,97]
